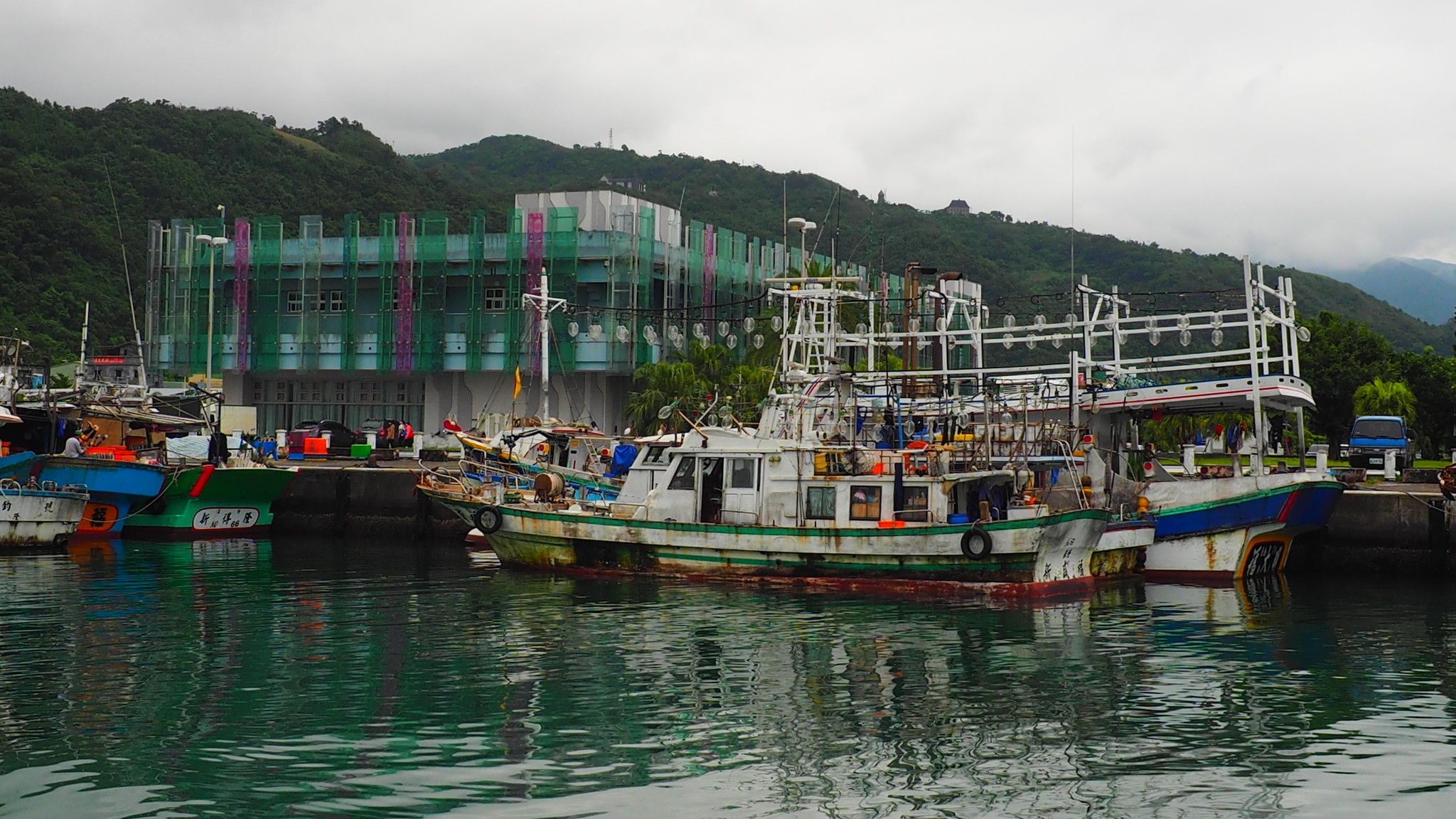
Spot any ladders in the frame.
[1051,438,1090,510]
[583,438,605,475]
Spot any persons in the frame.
[657,422,670,434]
[386,420,395,448]
[65,429,87,458]
[624,425,634,435]
[398,419,406,447]
[978,478,994,504]
[402,422,414,448]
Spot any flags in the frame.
[514,365,522,400]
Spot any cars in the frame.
[291,419,357,448]
[1304,443,1329,459]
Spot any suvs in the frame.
[355,417,406,450]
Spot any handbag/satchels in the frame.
[407,428,414,439]
[398,429,406,436]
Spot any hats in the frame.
[74,429,82,434]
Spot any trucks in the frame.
[1339,413,1418,473]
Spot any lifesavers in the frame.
[902,441,931,473]
[473,504,503,535]
[960,527,992,561]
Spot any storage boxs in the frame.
[254,430,371,460]
[85,445,136,462]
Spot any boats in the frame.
[413,196,1349,611]
[0,284,303,557]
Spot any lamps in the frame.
[568,315,580,337]
[692,316,710,349]
[1002,403,1011,428]
[668,319,684,348]
[718,312,737,349]
[590,316,629,343]
[642,317,657,346]
[784,408,795,432]
[838,408,847,435]
[957,402,968,429]
[904,409,915,438]
[539,313,550,333]
[657,400,678,419]
[872,414,883,442]
[707,405,733,431]
[1030,385,1042,408]
[744,307,1310,356]
[1053,389,1100,414]
